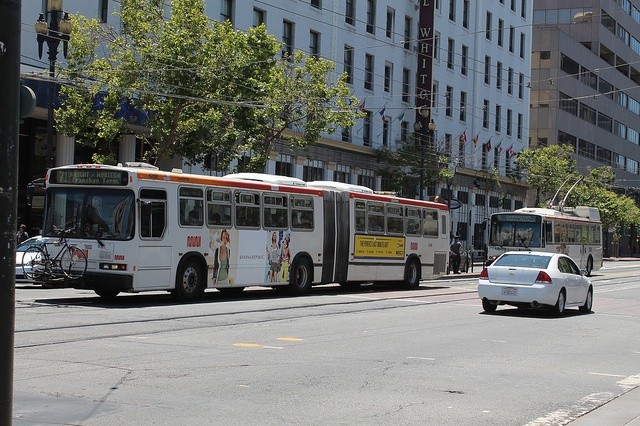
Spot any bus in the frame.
[42,162,450,301]
[487,175,603,277]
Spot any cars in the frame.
[15,235,42,276]
[478,251,593,316]
[449,244,472,273]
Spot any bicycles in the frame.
[22,225,88,280]
[430,195,462,210]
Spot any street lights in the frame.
[415,106,436,200]
[35,0,72,170]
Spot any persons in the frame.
[449,236,462,275]
[212,229,231,287]
[281,239,289,284]
[18,224,29,244]
[268,231,280,284]
